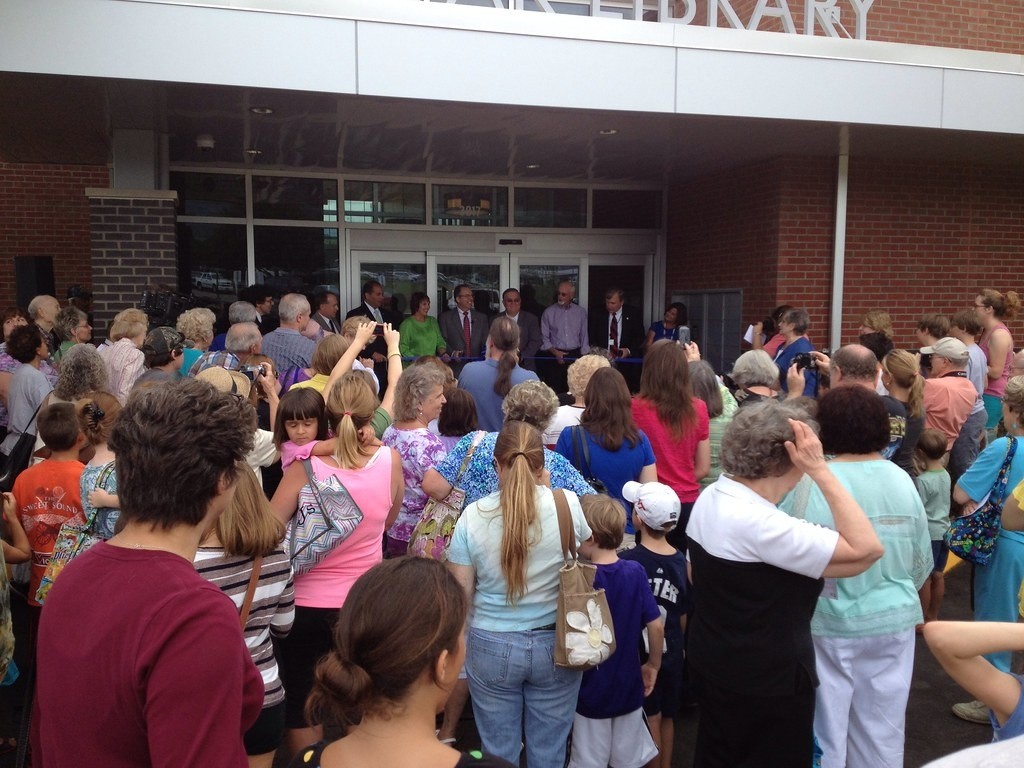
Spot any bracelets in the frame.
[387,351,402,358]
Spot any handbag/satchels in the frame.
[278,457,366,578]
[571,425,609,497]
[33,458,118,605]
[0,402,42,493]
[406,429,489,564]
[942,434,1017,566]
[552,488,616,671]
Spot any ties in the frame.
[374,310,383,323]
[610,312,619,359]
[329,319,336,334]
[463,312,471,357]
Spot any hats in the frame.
[194,367,251,405]
[142,326,195,356]
[622,480,681,532]
[919,336,970,359]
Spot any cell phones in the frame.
[372,324,384,336]
[679,328,691,350]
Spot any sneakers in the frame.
[952,700,991,724]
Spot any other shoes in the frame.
[914,621,930,632]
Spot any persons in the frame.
[0,279,1024,768]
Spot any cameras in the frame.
[918,353,932,368]
[241,365,265,381]
[790,352,818,369]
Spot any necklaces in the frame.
[115,534,183,555]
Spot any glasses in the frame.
[169,332,185,355]
[505,299,520,303]
[1008,365,1024,372]
[928,354,944,359]
[557,291,566,297]
[458,294,475,298]
[973,302,988,309]
[76,324,89,329]
[999,395,1008,406]
